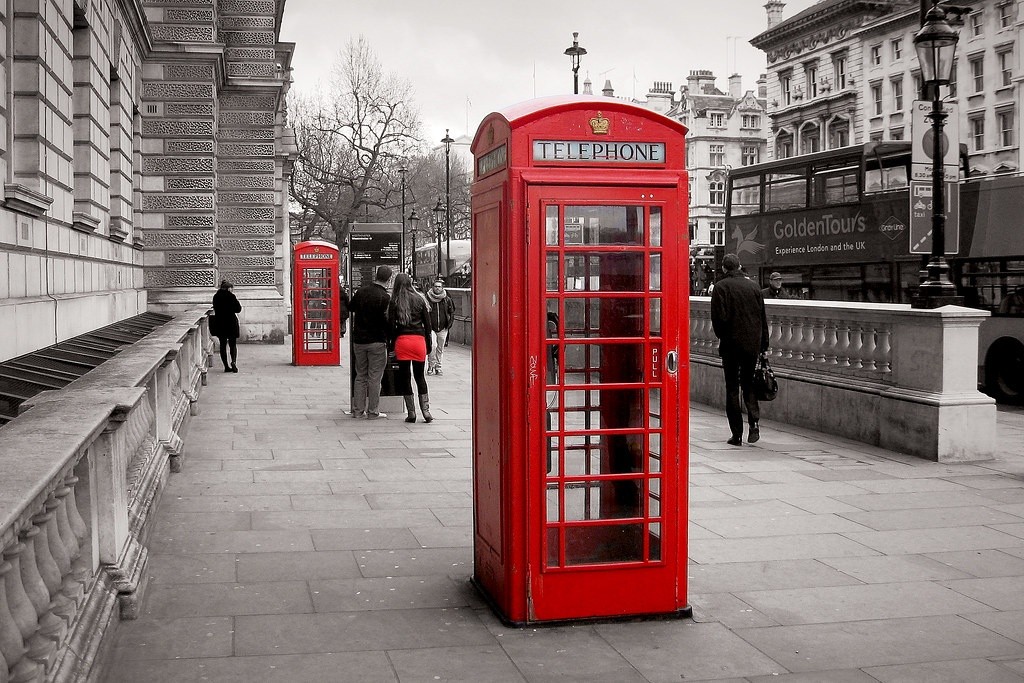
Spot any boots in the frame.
[404,394,416,423]
[418,393,433,422]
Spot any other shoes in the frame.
[231,362,238,373]
[368,413,387,419]
[225,368,232,372]
[435,364,443,376]
[355,410,367,418]
[428,365,433,375]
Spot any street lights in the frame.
[564,31,587,95]
[912,1,967,310]
[397,166,409,274]
[440,128,455,288]
[407,208,421,288]
[431,196,446,285]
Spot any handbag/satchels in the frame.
[209,316,217,336]
[752,353,778,401]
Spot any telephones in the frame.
[546,312,566,385]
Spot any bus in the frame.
[722,137,1023,313]
[343,221,405,298]
[407,240,471,288]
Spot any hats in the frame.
[770,272,782,279]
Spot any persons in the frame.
[759,270,790,299]
[213,280,241,373]
[712,254,769,447]
[997,284,1024,315]
[304,266,455,423]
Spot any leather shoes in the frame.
[727,437,742,447]
[748,423,759,443]
